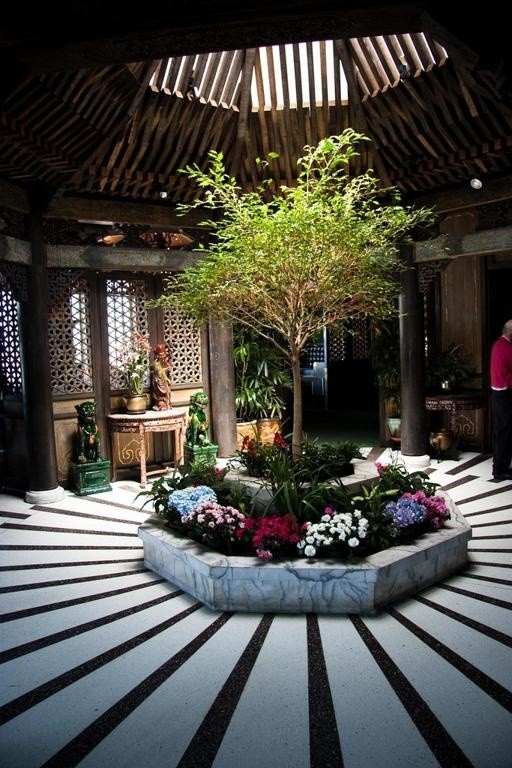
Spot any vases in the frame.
[122,393,149,415]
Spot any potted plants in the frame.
[232,324,293,452]
[367,333,400,438]
[425,339,482,391]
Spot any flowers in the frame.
[115,326,153,395]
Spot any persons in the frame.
[150,342,175,411]
[487,318,512,480]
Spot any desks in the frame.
[105,406,187,489]
[421,388,491,462]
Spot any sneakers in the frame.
[493,466,512,481]
